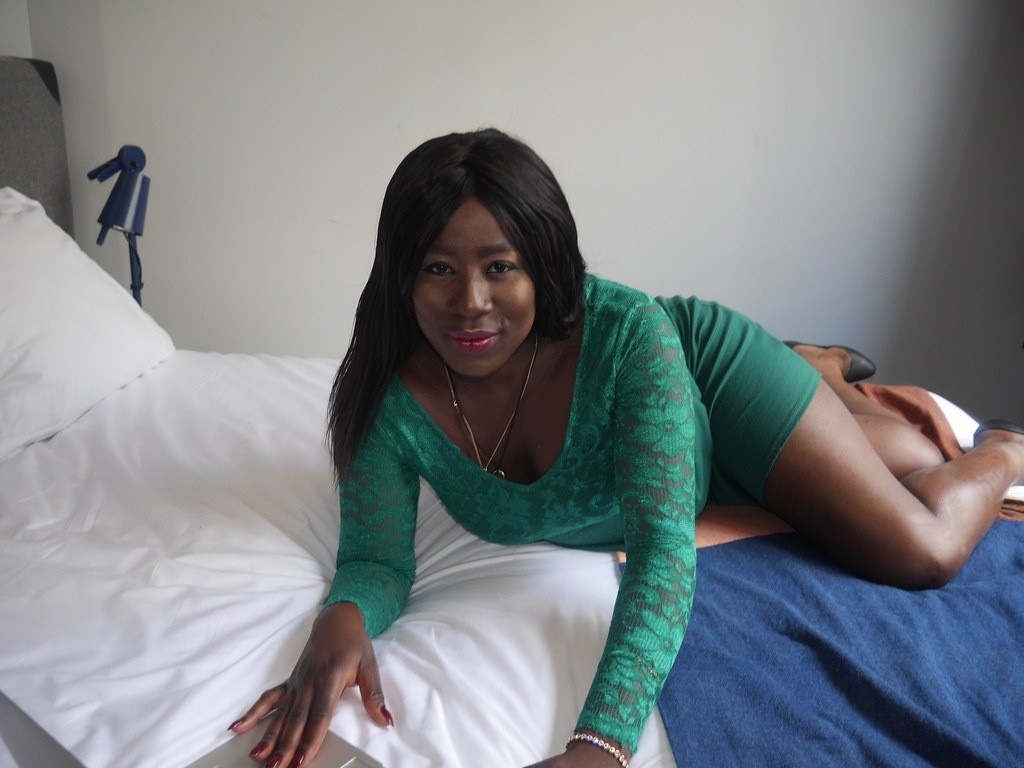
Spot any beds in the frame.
[1,350,1023,768]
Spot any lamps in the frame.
[87,145,150,308]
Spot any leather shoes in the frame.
[782,341,875,383]
[974,418,1024,449]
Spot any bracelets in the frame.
[565,734,630,768]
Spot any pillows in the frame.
[1,186,176,465]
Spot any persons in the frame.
[227,128,1024,768]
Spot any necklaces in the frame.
[444,334,539,478]
